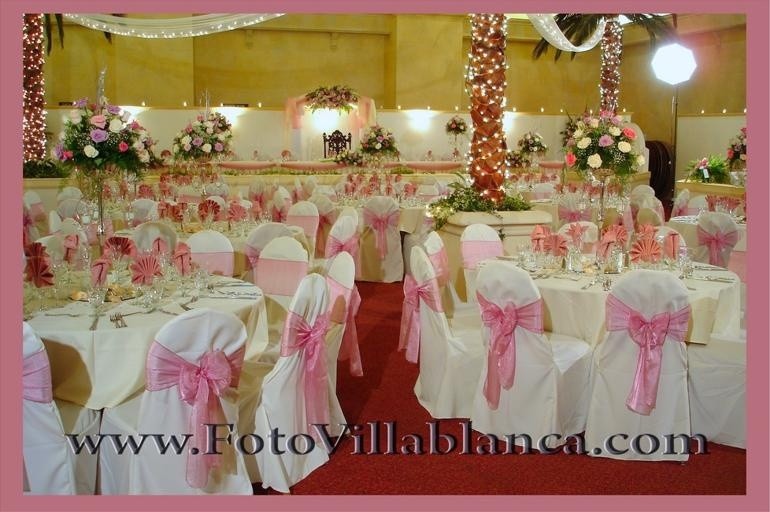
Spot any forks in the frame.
[111,313,130,329]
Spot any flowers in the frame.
[507,128,549,170]
[54,95,167,235]
[170,90,238,162]
[359,123,400,160]
[442,115,469,148]
[305,83,360,115]
[558,103,642,222]
[686,127,746,184]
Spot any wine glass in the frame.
[549,187,631,210]
[335,190,427,210]
[156,205,273,235]
[513,236,696,284]
[30,244,208,312]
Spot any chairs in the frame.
[23,172,746,496]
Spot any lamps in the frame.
[651,41,698,200]
